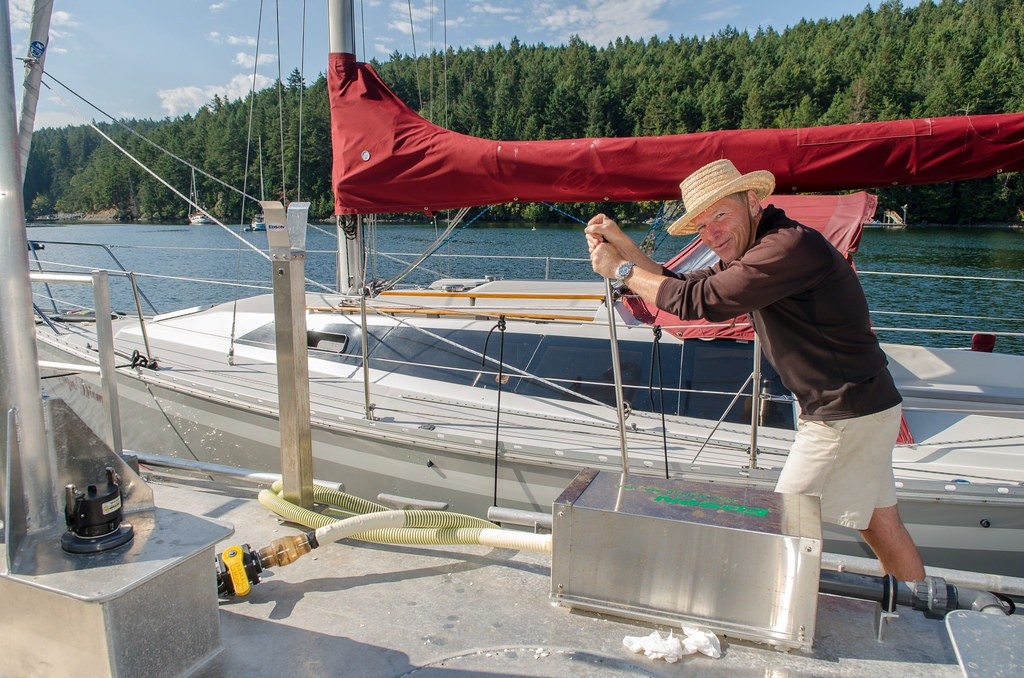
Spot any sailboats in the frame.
[244,135,268,231]
[0,0,1021,584]
[186,167,217,224]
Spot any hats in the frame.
[667,159,775,236]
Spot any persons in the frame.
[584,159,926,581]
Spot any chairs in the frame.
[971,334,997,351]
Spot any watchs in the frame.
[617,262,636,284]
